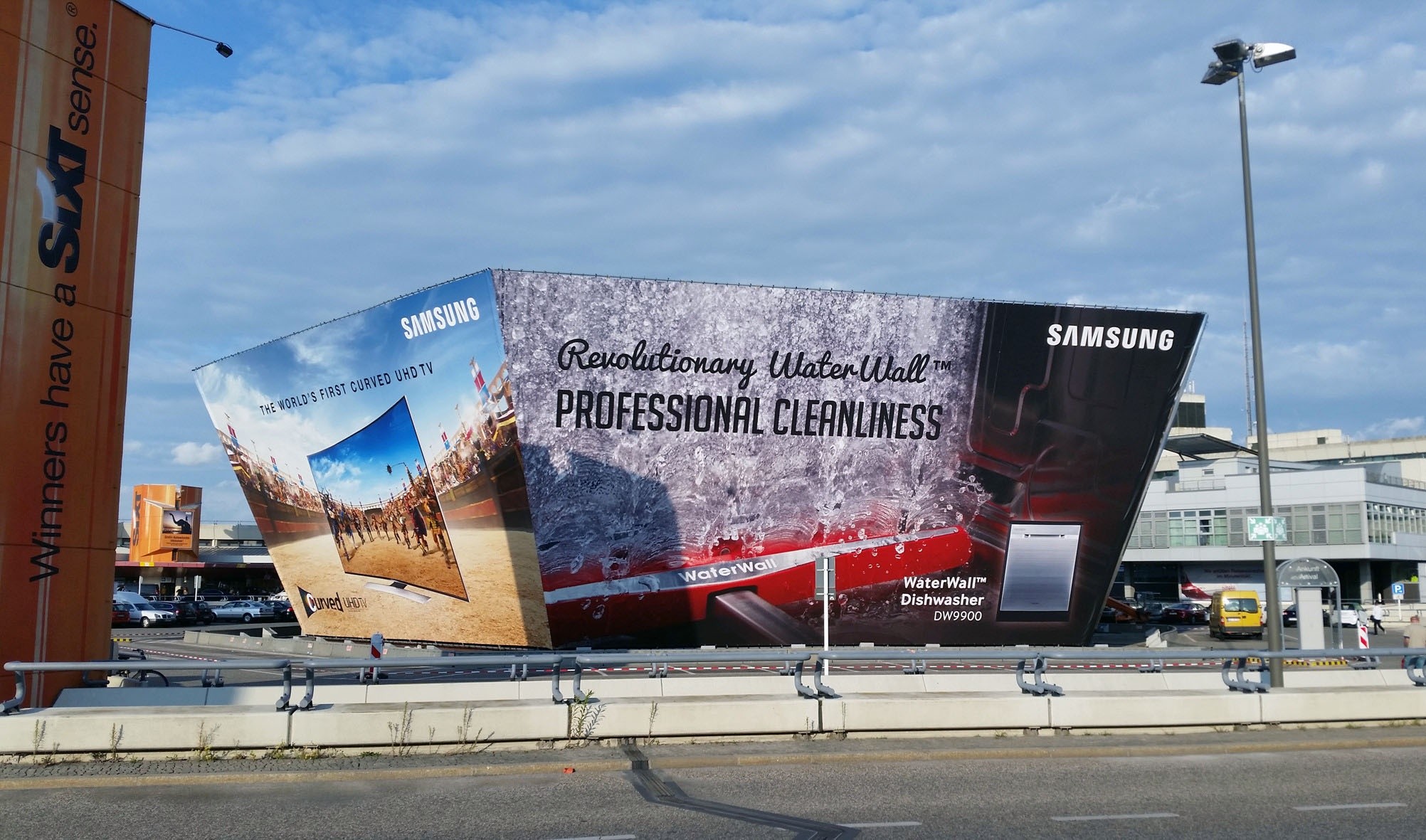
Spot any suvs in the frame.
[1330,602,1370,627]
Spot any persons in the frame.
[1403,616,1426,668]
[326,496,451,569]
[1371,600,1386,636]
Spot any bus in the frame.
[1204,591,1263,640]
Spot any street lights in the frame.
[1197,38,1296,685]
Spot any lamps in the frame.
[153,20,233,58]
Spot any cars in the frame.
[1161,603,1210,625]
[1283,603,1330,628]
[112,585,296,627]
[1101,599,1165,623]
[1259,604,1268,626]
